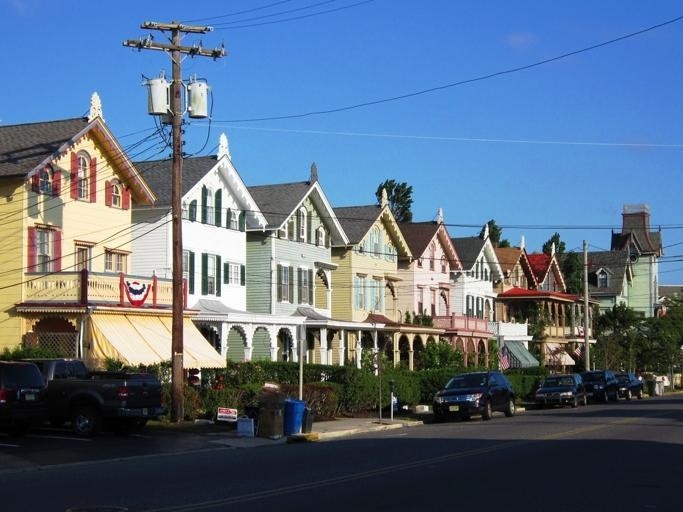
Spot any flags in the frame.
[572,344,585,359]
[494,344,511,371]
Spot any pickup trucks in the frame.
[21,356,172,438]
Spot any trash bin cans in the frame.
[256,397,314,439]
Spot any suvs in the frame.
[533,368,645,408]
[0,358,49,434]
[431,370,514,421]
